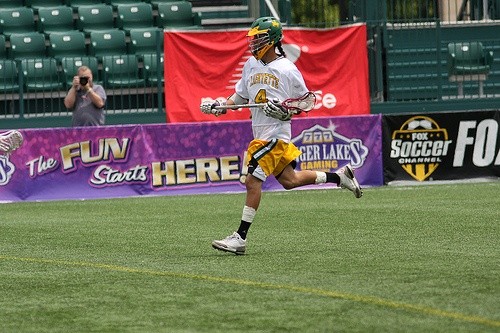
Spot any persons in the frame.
[64,65,107,126]
[201,16,363,256]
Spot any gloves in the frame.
[262,97,293,122]
[199,97,228,115]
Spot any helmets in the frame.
[246,16,284,60]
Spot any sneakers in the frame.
[336,165,363,198]
[212,232,247,256]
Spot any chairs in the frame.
[0,0,203,105]
[448,41,493,96]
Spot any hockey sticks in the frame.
[210,92,317,113]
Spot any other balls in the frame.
[298,102,307,108]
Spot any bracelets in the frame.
[86,88,92,94]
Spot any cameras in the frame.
[80,77,88,85]
